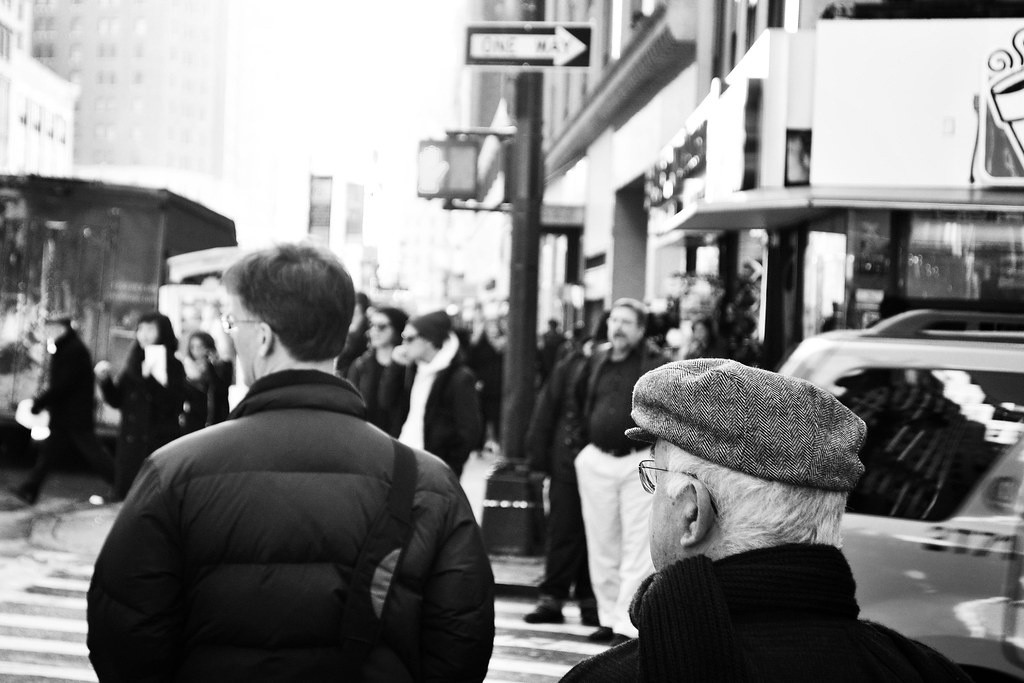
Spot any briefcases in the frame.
[480,459,551,565]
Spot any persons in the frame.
[554,358,1023,683]
[7,293,720,643]
[85,243,495,683]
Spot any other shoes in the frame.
[522,604,565,624]
[7,482,39,506]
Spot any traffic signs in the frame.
[462,19,598,71]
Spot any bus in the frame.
[1,176,237,438]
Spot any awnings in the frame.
[657,191,819,235]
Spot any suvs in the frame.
[776,309,1024,683]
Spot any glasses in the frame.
[639,460,698,493]
[367,318,390,331]
[220,310,262,332]
[400,333,420,343]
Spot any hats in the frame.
[623,357,868,490]
[376,307,409,335]
[411,309,453,349]
